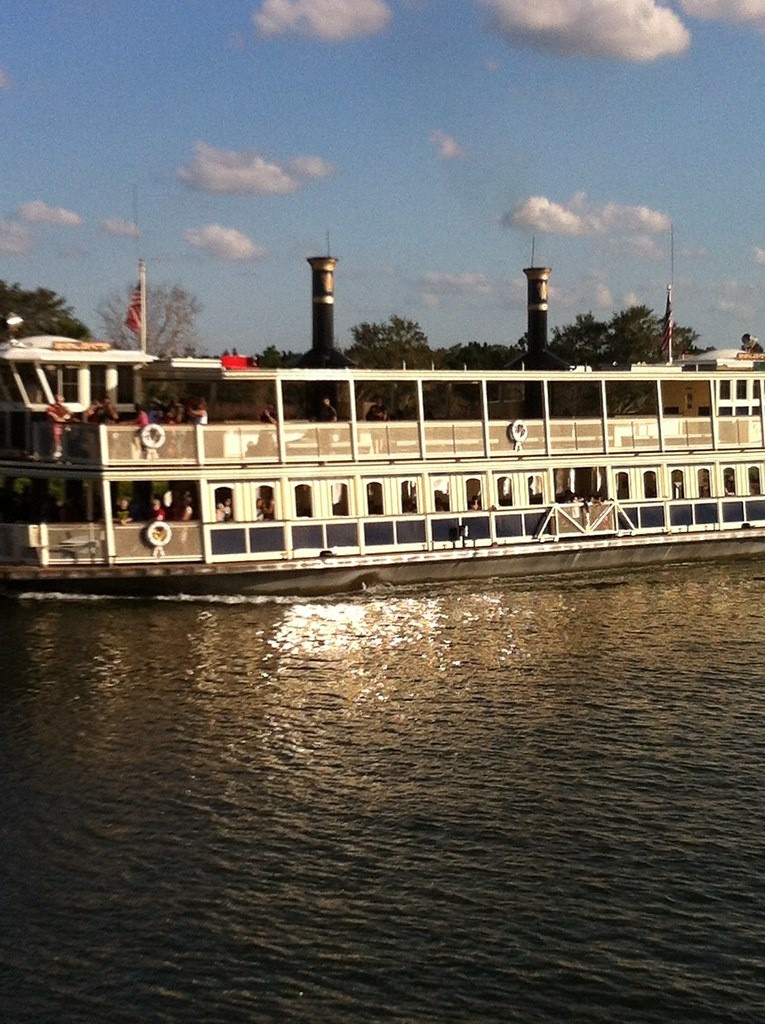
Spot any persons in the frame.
[318,399,337,422]
[257,404,278,425]
[189,401,208,425]
[147,498,166,524]
[256,497,274,521]
[115,495,134,524]
[179,492,195,522]
[216,498,232,521]
[24,380,78,459]
[365,395,387,421]
[85,395,186,424]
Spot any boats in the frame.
[0,256,765,597]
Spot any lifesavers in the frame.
[138,423,167,450]
[509,419,529,442]
[143,520,173,546]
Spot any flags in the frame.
[125,280,144,336]
[661,292,673,354]
